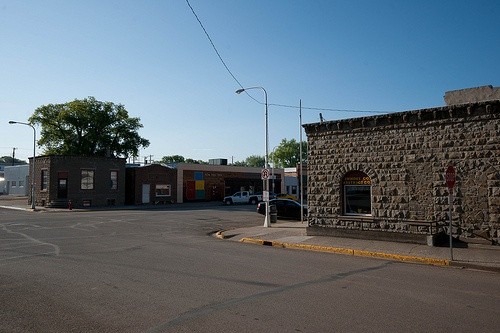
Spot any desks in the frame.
[103,197,116,209]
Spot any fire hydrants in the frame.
[68,200,72,210]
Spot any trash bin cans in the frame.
[269,205,277,223]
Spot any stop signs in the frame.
[445,166,456,189]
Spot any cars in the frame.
[257,198,307,221]
[270,193,299,202]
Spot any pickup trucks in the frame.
[222,190,263,206]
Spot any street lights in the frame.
[9,120,36,210]
[235,86,271,228]
